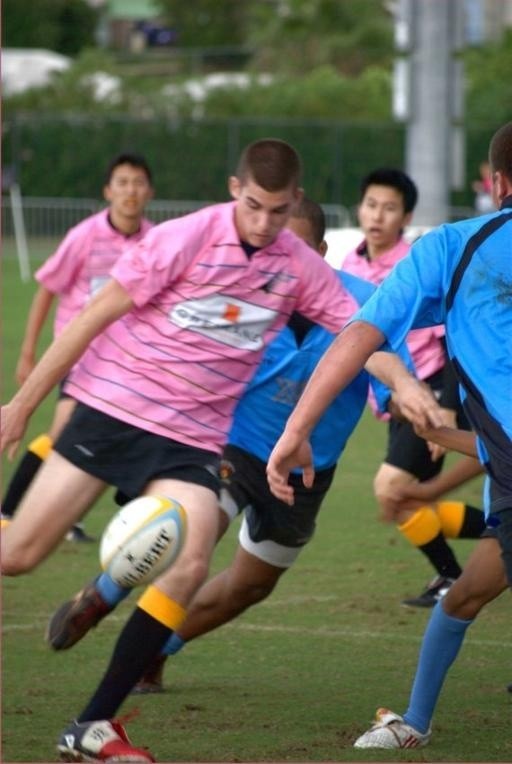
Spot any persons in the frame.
[1,156,158,542]
[46,122,512,750]
[1,137,449,763]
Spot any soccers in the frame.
[100,494,188,586]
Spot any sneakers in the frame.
[135,652,165,695]
[352,706,432,750]
[54,718,155,763]
[43,568,118,652]
[401,574,457,612]
[66,522,86,543]
